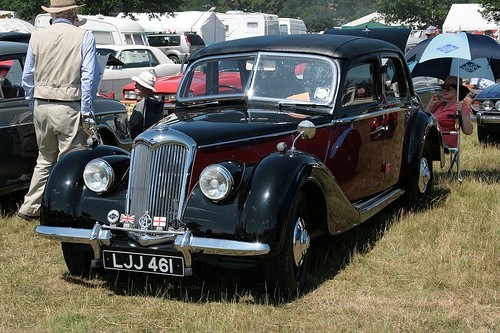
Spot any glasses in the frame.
[441,86,456,91]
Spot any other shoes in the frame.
[17,213,40,223]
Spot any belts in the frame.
[35,98,80,103]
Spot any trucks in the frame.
[3,7,309,96]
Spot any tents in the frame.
[115,11,226,46]
[334,4,500,38]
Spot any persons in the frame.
[426,76,474,148]
[128,70,164,141]
[17,0,100,221]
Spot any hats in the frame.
[41,0,85,13]
[130,71,157,92]
[432,76,470,101]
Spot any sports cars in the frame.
[117,52,252,112]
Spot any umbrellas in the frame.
[385,30,500,128]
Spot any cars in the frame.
[471,81,500,144]
[32,32,445,304]
[410,77,444,106]
[1,40,129,207]
